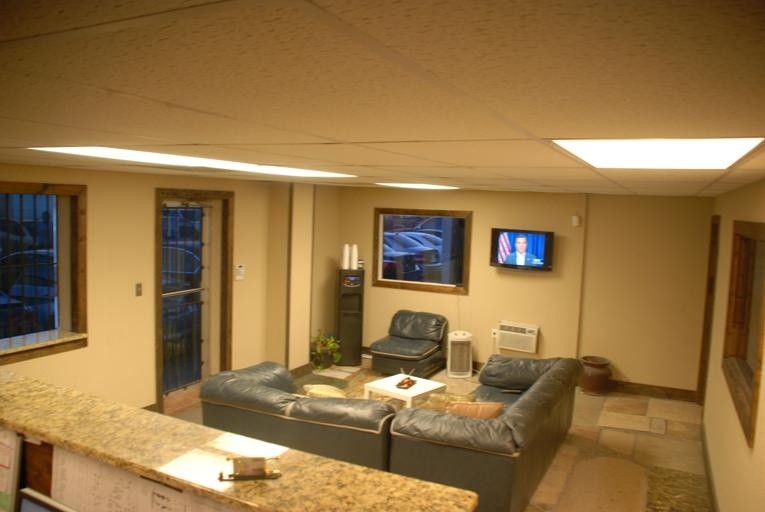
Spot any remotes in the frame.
[501,389,522,394]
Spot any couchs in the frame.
[391,355,579,507]
[206,358,389,466]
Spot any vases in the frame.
[582,353,613,395]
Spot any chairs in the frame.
[368,308,448,382]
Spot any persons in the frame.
[501,233,541,267]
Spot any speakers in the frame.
[447,331,473,377]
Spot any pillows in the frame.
[427,393,506,423]
[301,382,344,400]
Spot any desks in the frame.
[365,369,446,403]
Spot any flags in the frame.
[497,231,512,266]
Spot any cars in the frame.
[383,228,442,279]
[1,218,201,359]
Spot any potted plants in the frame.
[309,328,343,371]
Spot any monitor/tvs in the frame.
[490,228,554,272]
